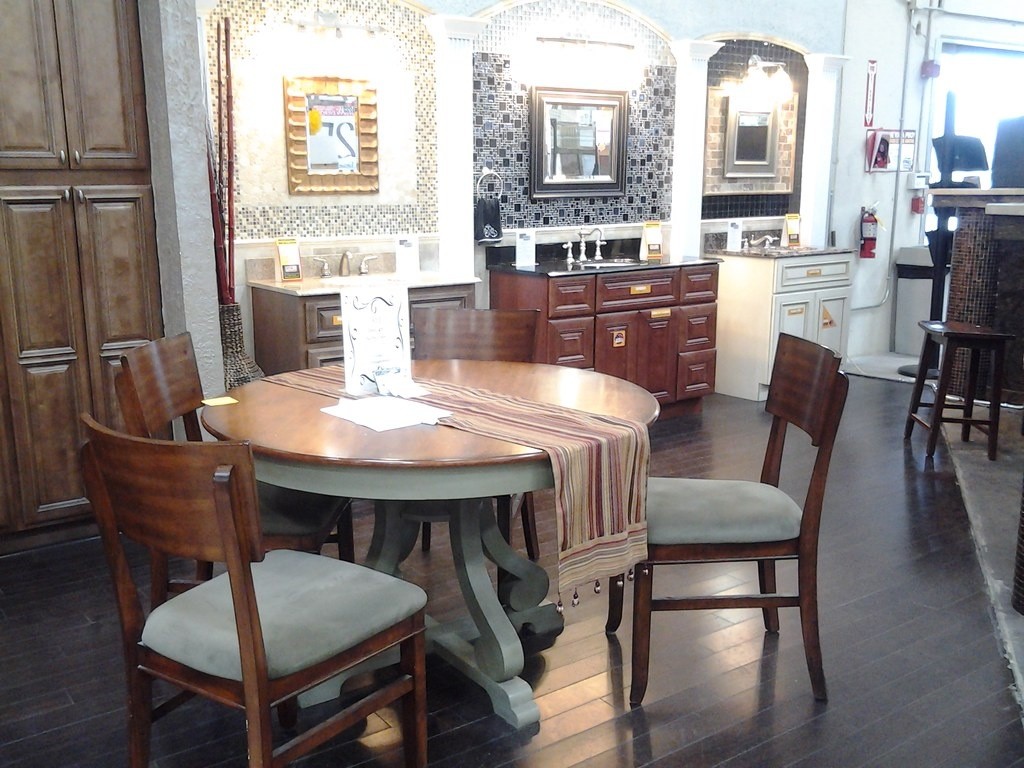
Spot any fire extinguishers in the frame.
[861,202,878,259]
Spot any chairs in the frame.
[113,330,356,614]
[410,304,540,590]
[602,331,851,711]
[72,411,430,768]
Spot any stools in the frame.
[902,319,1016,462]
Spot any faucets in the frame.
[749,234,779,249]
[578,228,605,261]
[337,250,353,276]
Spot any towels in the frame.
[474,196,503,246]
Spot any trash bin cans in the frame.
[895,245,951,358]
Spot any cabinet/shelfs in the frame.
[252,284,475,376]
[0,0,175,560]
[489,265,720,421]
[716,252,857,403]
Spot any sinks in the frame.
[754,247,818,253]
[571,259,649,268]
[321,275,408,286]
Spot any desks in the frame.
[200,359,662,734]
[929,186,1024,408]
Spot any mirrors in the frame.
[704,86,800,198]
[284,75,382,196]
[530,85,629,195]
